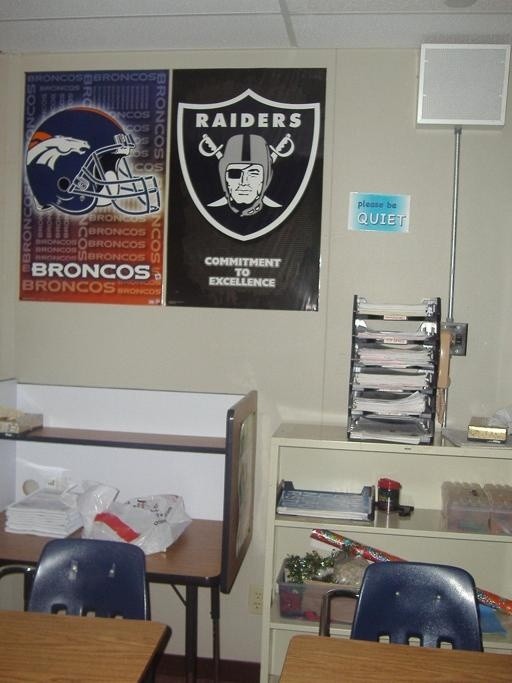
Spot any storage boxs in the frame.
[440,481,512,537]
[276,558,359,627]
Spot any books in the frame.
[4,487,85,539]
[276,487,372,521]
[0,410,45,436]
[349,299,435,444]
[439,428,512,449]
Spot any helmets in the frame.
[24,105,136,218]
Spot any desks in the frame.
[2,504,227,682]
[1,610,173,682]
[277,633,511,683]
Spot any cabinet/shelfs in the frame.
[259,419,511,682]
[1,375,257,596]
[346,292,440,443]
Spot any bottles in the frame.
[377,478,400,511]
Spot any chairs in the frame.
[350,560,483,652]
[20,540,151,624]
